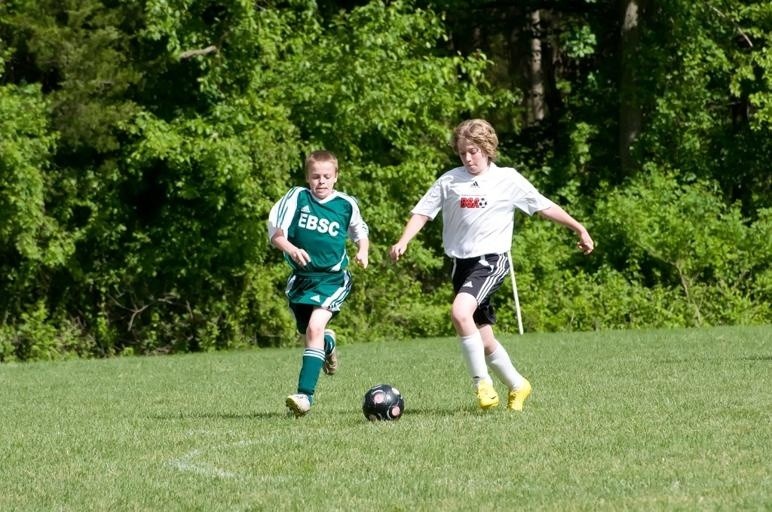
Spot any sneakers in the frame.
[322,329,337,376]
[507,378,532,411]
[286,394,311,415]
[475,381,499,411]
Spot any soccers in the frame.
[363,385,404,422]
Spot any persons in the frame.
[389,119,594,413]
[267,151,369,414]
